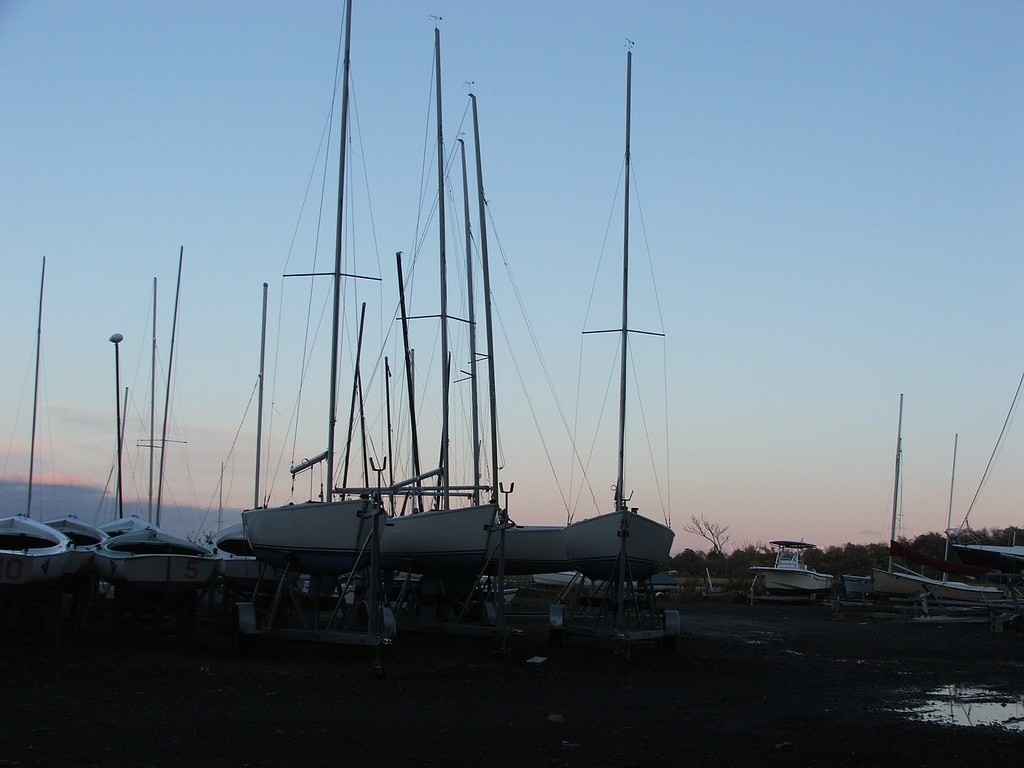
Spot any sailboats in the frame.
[872,371,1024,604]
[0,0,681,610]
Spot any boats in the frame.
[842,574,872,595]
[749,540,835,597]
